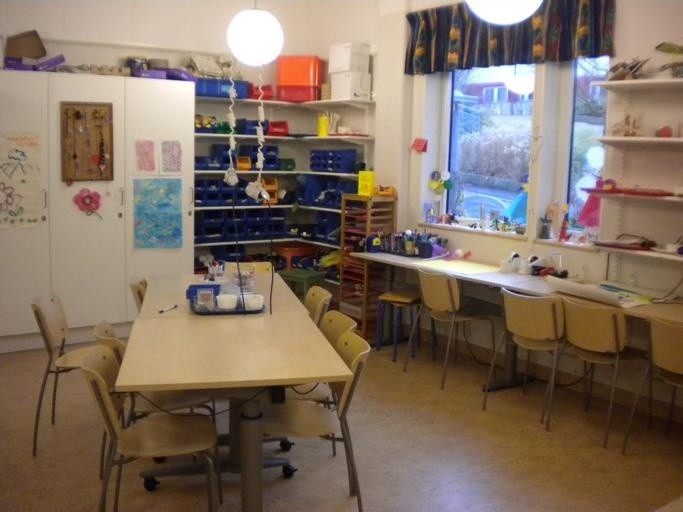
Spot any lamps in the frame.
[464,0,545,27]
[225,0,283,67]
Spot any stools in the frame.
[377,289,436,362]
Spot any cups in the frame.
[216,294,237,310]
[240,294,263,311]
[317,116,330,137]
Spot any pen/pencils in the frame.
[376,228,448,249]
[324,112,340,132]
[207,262,224,272]
[159,304,177,314]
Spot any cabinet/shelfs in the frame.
[340,194,397,345]
[583,78,683,262]
[0,69,194,353]
[194,95,377,289]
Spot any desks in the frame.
[112,273,355,512]
[349,251,542,391]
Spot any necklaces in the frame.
[64,106,109,186]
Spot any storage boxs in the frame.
[327,43,370,73]
[331,71,372,98]
[196,78,248,98]
[276,54,324,102]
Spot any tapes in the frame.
[528,254,539,262]
[508,252,520,259]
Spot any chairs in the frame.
[621,317,683,453]
[302,285,332,327]
[80,349,220,512]
[93,320,218,479]
[403,267,495,391]
[544,296,655,450]
[93,320,127,356]
[286,310,357,457]
[261,332,371,512]
[482,287,590,432]
[32,291,92,458]
[129,278,149,311]
